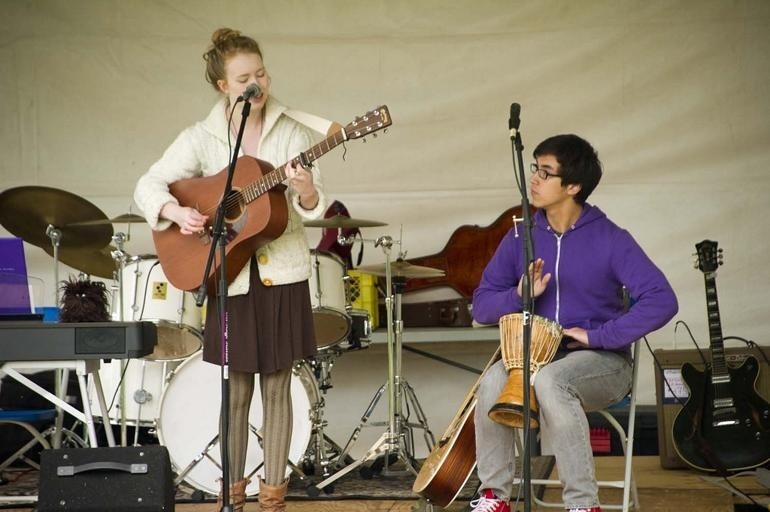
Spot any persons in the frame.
[473,133,677,512]
[134,25,327,512]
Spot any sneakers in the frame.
[470,488,511,512]
[568,508,602,512]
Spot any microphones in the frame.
[508,101,521,141]
[237,83,261,103]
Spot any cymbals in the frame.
[303,214,388,228]
[43,246,131,280]
[1,185,114,254]
[69,212,147,230]
[358,259,446,278]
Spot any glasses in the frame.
[529,163,562,180]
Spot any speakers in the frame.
[75,326,127,354]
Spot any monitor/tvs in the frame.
[0,236,44,321]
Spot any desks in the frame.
[371,322,506,344]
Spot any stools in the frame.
[2,360,119,507]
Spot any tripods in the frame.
[331,277,437,482]
[306,235,423,497]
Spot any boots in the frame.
[258,475,290,512]
[218,477,246,512]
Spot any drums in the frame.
[86,358,183,428]
[111,254,204,362]
[308,247,352,350]
[326,306,373,356]
[488,313,565,429]
[155,350,326,500]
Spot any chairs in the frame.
[512,285,639,512]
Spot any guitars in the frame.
[151,105,392,293]
[412,344,502,508]
[671,240,770,472]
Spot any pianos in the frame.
[0,321,158,361]
